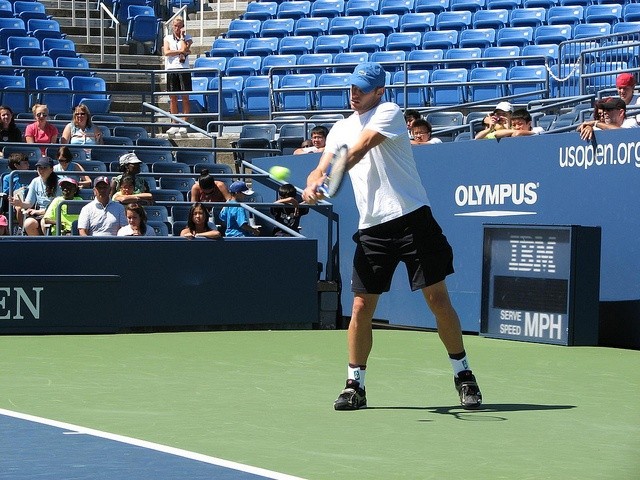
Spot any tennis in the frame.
[270,165,291,181]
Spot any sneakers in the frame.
[454,370,482,409]
[334,379,367,410]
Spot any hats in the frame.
[94,176,109,188]
[119,153,142,166]
[228,180,255,196]
[349,63,386,94]
[616,72,636,88]
[494,101,514,114]
[34,157,54,169]
[596,98,626,112]
[58,178,78,188]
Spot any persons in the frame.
[0,104,25,142]
[291,126,330,154]
[301,140,313,149]
[181,204,220,239]
[10,156,64,235]
[29,103,60,146]
[577,95,639,142]
[59,103,106,146]
[0,213,11,234]
[404,109,419,137]
[51,145,94,191]
[411,120,445,145]
[40,177,86,239]
[164,18,193,122]
[1,152,32,198]
[219,181,263,237]
[112,171,154,202]
[115,154,149,194]
[615,74,640,120]
[190,167,226,207]
[269,182,306,239]
[474,101,513,138]
[116,202,156,240]
[77,174,125,238]
[494,108,547,137]
[303,60,482,409]
[593,100,606,122]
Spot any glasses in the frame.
[36,113,47,117]
[75,113,87,115]
[59,160,69,163]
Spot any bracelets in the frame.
[594,121,600,127]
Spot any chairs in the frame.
[186,190,194,205]
[520,43,559,66]
[226,55,260,74]
[259,17,293,37]
[363,13,399,35]
[171,205,192,220]
[158,176,195,192]
[535,121,550,132]
[436,11,470,27]
[144,178,155,188]
[225,19,260,38]
[17,112,36,119]
[241,75,281,112]
[549,64,586,97]
[0,55,17,74]
[99,126,110,135]
[89,149,126,162]
[207,74,242,114]
[109,161,148,172]
[392,69,428,105]
[56,114,75,118]
[430,67,467,104]
[191,58,224,74]
[136,138,173,148]
[585,3,621,24]
[211,38,247,56]
[482,46,520,69]
[238,123,276,163]
[273,115,307,130]
[574,103,592,118]
[349,32,386,51]
[347,1,380,15]
[261,54,297,75]
[328,16,363,34]
[295,16,329,34]
[143,205,169,222]
[276,35,315,56]
[19,55,54,75]
[472,9,508,29]
[18,172,37,183]
[167,75,208,114]
[243,36,277,55]
[92,115,125,122]
[6,36,40,54]
[572,21,612,42]
[613,20,640,42]
[623,3,640,21]
[456,28,495,48]
[551,121,572,129]
[333,52,369,72]
[151,162,192,174]
[46,148,87,160]
[0,74,29,112]
[315,72,352,107]
[416,0,447,13]
[35,75,72,114]
[524,0,552,8]
[278,73,315,110]
[380,0,413,14]
[2,159,45,171]
[400,11,435,32]
[445,48,480,70]
[312,1,346,18]
[560,41,599,65]
[384,32,422,52]
[314,33,350,54]
[3,145,41,159]
[506,64,549,100]
[43,38,76,57]
[279,124,318,156]
[560,113,577,119]
[421,29,457,49]
[0,1,16,16]
[14,0,48,18]
[193,163,233,183]
[585,62,627,94]
[371,51,407,68]
[148,220,171,236]
[466,111,492,126]
[311,114,346,127]
[426,110,464,142]
[128,4,158,41]
[541,115,557,121]
[54,123,67,133]
[71,75,109,113]
[496,26,532,45]
[104,137,132,146]
[175,150,214,162]
[135,149,176,162]
[113,126,147,138]
[533,24,574,45]
[467,66,508,101]
[243,2,276,20]
[601,41,640,62]
[172,221,189,236]
[408,48,442,69]
[545,5,584,25]
[71,219,80,236]
[509,8,545,27]
[488,1,521,10]
[75,160,107,178]
[56,57,97,78]
[454,131,475,142]
[25,18,61,37]
[0,17,25,36]
[278,1,309,19]
[295,53,331,74]
[381,72,392,106]
[561,0,589,7]
[320,121,333,130]
[252,207,274,229]
[152,189,186,202]
[18,122,30,131]
[451,0,484,11]
